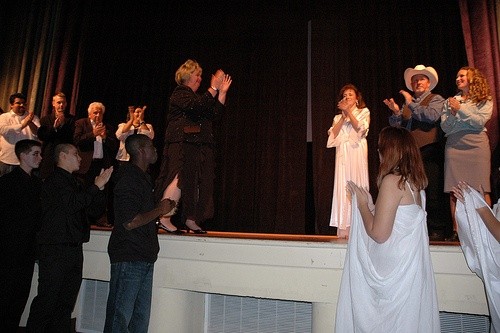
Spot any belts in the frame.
[92,158,103,162]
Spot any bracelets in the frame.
[140,122,145,125]
[210,86,218,91]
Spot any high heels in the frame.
[157,222,183,235]
[185,224,207,234]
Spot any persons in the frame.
[326,84,370,238]
[334,127,440,333]
[0,58,232,333]
[453,180,500,333]
[383,65,445,240]
[440,66,492,240]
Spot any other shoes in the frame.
[97,220,113,227]
[429,230,444,241]
[444,231,459,241]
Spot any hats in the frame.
[404,64,438,92]
